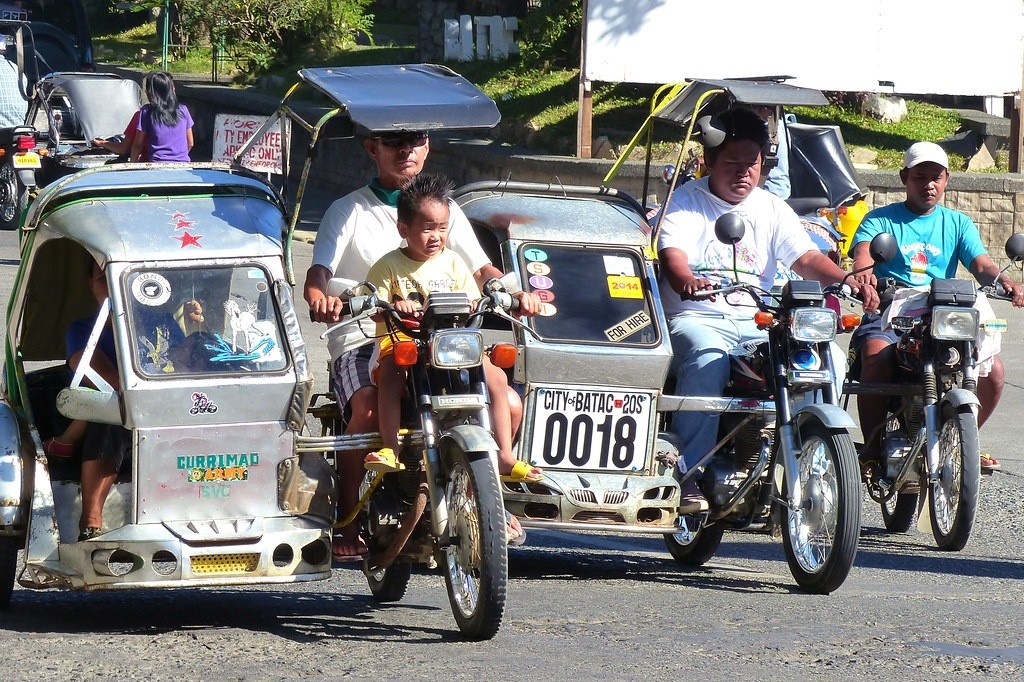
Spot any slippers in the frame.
[332,531,372,562]
[364,448,406,472]
[500,460,544,482]
[980,453,1001,468]
[78,526,103,542]
[859,459,878,471]
[505,510,526,545]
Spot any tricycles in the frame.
[663,113,869,334]
[447,80,898,594]
[0,64,545,641]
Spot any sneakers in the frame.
[678,474,709,514]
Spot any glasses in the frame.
[374,131,428,149]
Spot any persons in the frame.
[654,106,881,518]
[37,253,250,543]
[90,70,195,162]
[848,138,1024,496]
[303,128,545,563]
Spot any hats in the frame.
[906,142,948,173]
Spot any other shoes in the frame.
[43,437,73,457]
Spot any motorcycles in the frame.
[0,125,48,231]
[87,135,130,164]
[842,231,1024,553]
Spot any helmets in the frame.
[0,33,6,50]
